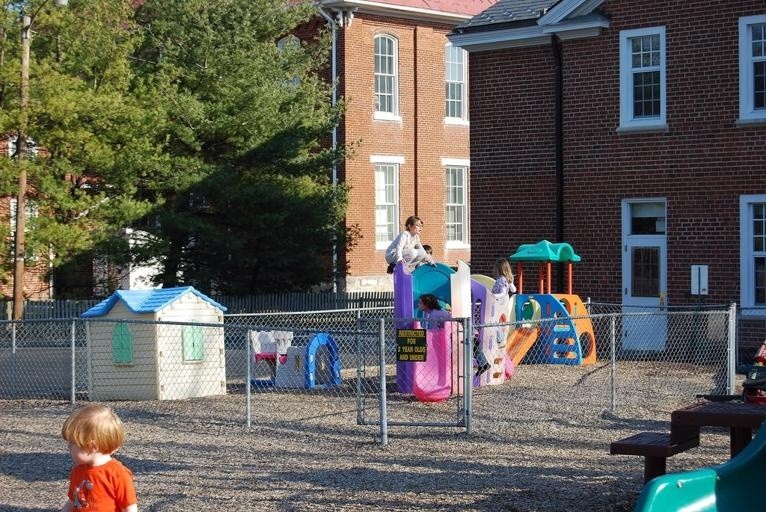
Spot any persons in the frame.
[62,405,138,512]
[418,294,491,377]
[385,216,436,273]
[491,258,516,294]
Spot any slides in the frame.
[636,426,762,512]
[507,326,540,365]
[412,321,464,401]
